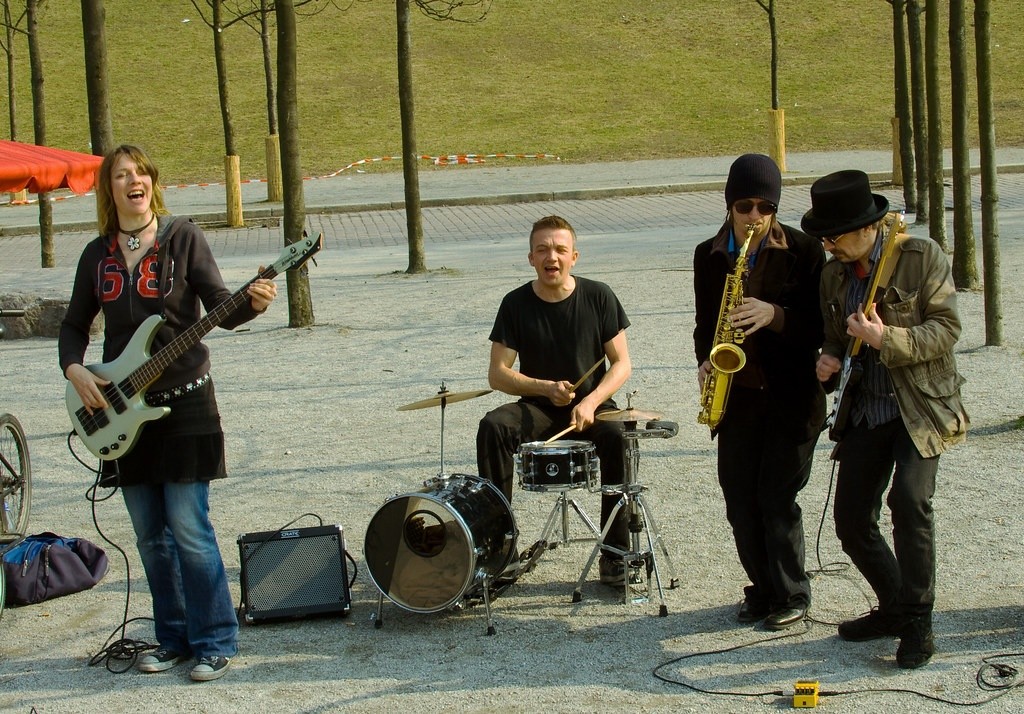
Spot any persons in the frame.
[476,214,641,584]
[58,146,280,682]
[801,170,972,670]
[692,154,829,629]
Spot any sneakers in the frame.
[897,615,936,668]
[838,609,898,642]
[191,657,232,680]
[504,547,520,572]
[139,648,188,671]
[599,559,636,582]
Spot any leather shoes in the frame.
[737,592,811,630]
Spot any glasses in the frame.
[815,234,845,245]
[734,200,777,215]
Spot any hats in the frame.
[801,170,889,236]
[725,153,782,209]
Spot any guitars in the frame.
[64,230,324,461]
[827,213,906,442]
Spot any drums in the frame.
[516,441,599,493]
[363,472,518,613]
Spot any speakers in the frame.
[238,523,352,623]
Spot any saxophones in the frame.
[698,223,758,430]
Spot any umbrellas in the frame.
[0,139,103,197]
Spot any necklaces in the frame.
[119,214,155,252]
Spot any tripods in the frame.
[526,437,682,618]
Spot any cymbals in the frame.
[595,409,665,423]
[395,388,494,412]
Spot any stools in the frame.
[538,490,600,547]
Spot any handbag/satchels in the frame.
[0,532,108,608]
